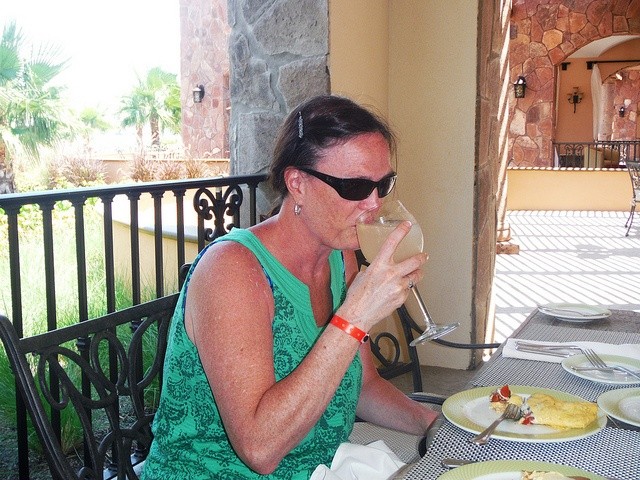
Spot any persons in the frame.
[137,94,444,479]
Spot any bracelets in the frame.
[329,314,370,344]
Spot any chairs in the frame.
[624,159,640,237]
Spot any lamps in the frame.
[615,71,623,82]
[567,87,584,114]
[619,106,625,117]
[513,76,526,98]
[193,84,202,103]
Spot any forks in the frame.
[580,348,640,379]
[468,396,526,443]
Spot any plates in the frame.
[441,385,608,444]
[597,386,640,428]
[436,460,610,480]
[561,353,640,385]
[539,302,611,324]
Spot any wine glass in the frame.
[356,198,460,347]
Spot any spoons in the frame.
[538,305,610,318]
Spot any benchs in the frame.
[0,246,503,479]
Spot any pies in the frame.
[524,393,599,430]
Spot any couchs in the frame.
[583,145,619,168]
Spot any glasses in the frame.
[299,169,397,201]
